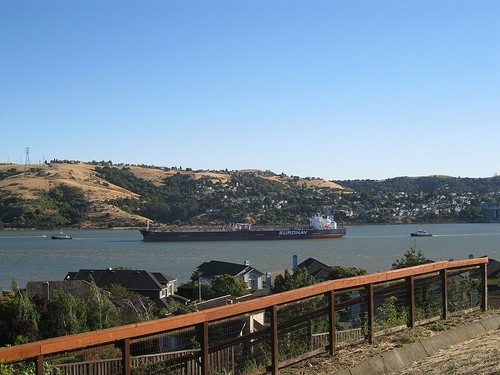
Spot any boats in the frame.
[410,229,435,237]
[51,230,73,240]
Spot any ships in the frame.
[140,211,346,242]
